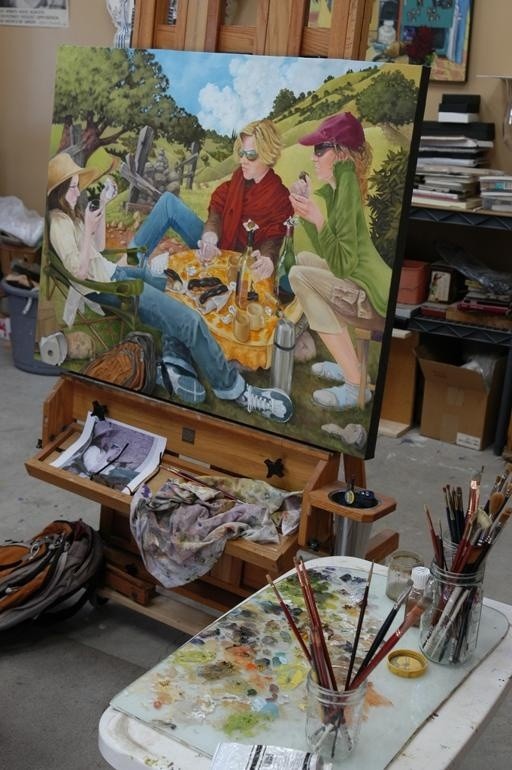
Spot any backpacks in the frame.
[79,331,177,397]
[1,518,108,645]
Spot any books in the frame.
[411,121,512,213]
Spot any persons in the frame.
[287,111,394,412]
[83,421,140,485]
[118,120,295,268]
[46,152,294,422]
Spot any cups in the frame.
[228,254,241,285]
[233,304,265,343]
[90,199,105,234]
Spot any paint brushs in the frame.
[423,463,512,663]
[268,554,425,760]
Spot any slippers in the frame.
[311,361,372,384]
[311,380,372,411]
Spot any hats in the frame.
[93,420,112,438]
[46,153,97,196]
[298,113,365,147]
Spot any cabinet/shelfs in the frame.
[24,377,399,638]
[380,197,512,456]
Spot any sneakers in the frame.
[156,362,205,405]
[235,383,294,423]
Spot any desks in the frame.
[99,557,512,769]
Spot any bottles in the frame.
[441,530,459,557]
[331,478,376,559]
[236,216,257,309]
[305,665,367,763]
[404,566,431,628]
[270,320,296,396]
[421,551,485,665]
[273,219,299,302]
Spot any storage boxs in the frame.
[411,345,502,452]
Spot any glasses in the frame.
[315,143,334,156]
[238,150,258,161]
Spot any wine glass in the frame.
[291,182,309,225]
[197,241,221,278]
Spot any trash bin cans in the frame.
[1,274,69,376]
